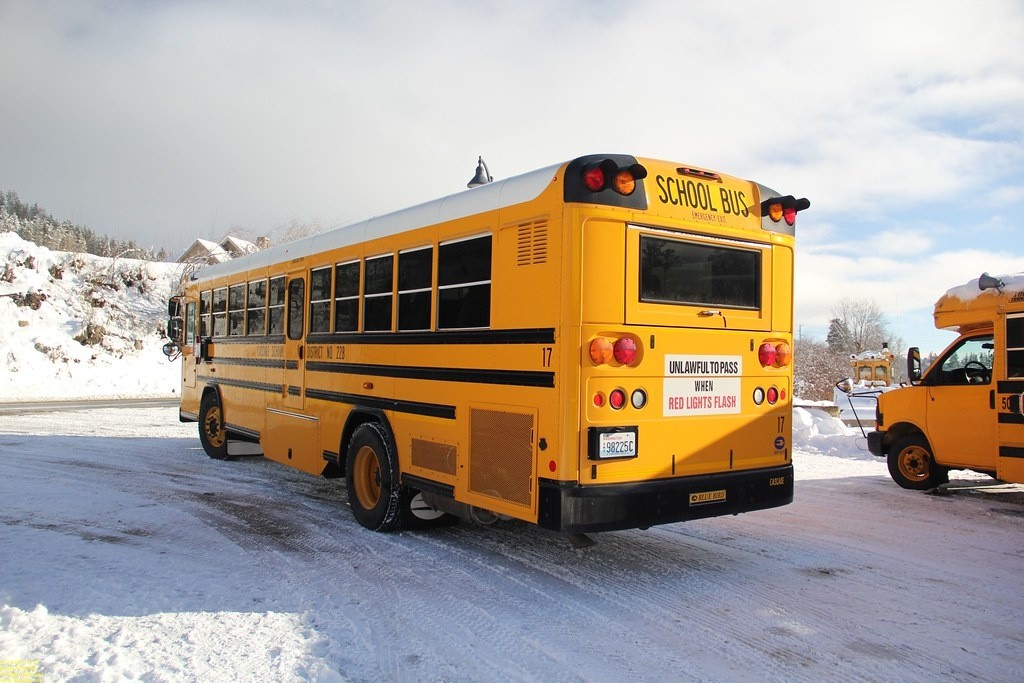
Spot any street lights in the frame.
[467,156,493,188]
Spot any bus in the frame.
[836,272,1024,490]
[162,154,810,549]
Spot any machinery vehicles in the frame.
[833,352,906,427]
